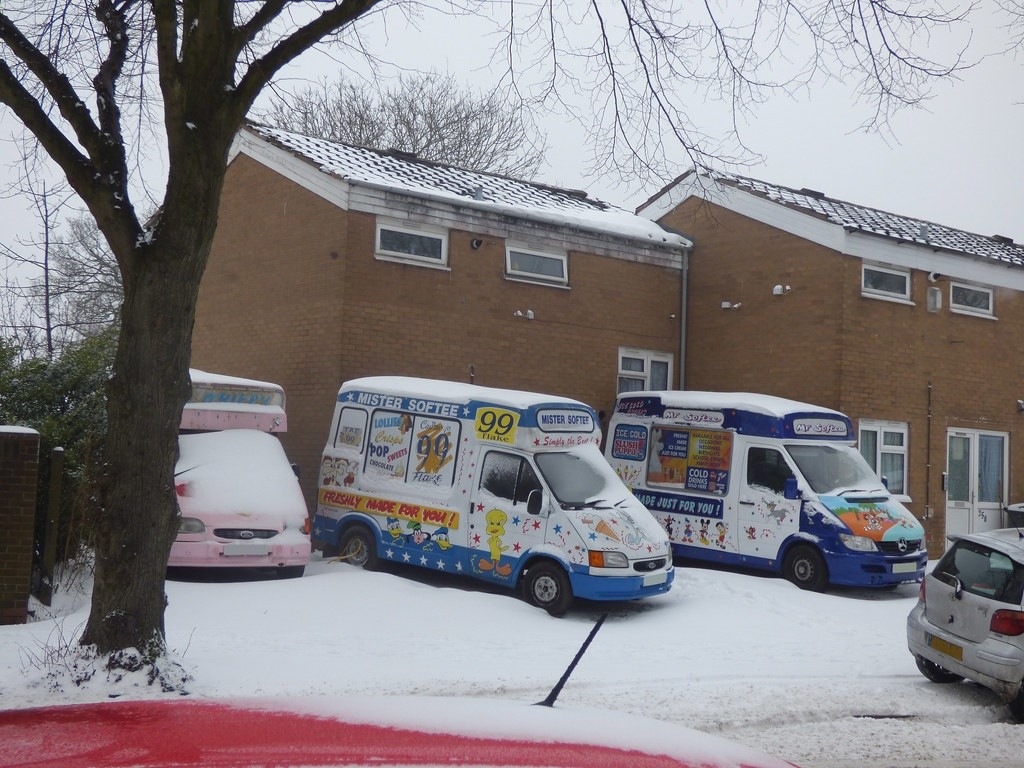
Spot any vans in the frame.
[308,376,676,618]
[603,387,929,594]
[166,367,311,581]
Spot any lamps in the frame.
[772,285,784,295]
[722,302,731,308]
[513,310,534,320]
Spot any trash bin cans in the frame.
[1003,502,1024,528]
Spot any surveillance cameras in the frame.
[471,239,482,249]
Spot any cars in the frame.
[906,528,1024,723]
